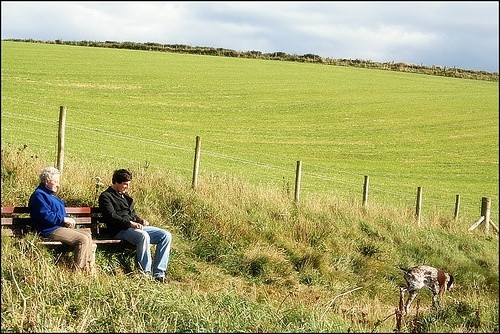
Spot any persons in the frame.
[28,166,98,281]
[98,169,172,283]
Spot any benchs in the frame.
[0,207,152,264]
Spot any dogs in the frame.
[398,264,455,316]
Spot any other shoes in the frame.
[155,275,166,283]
